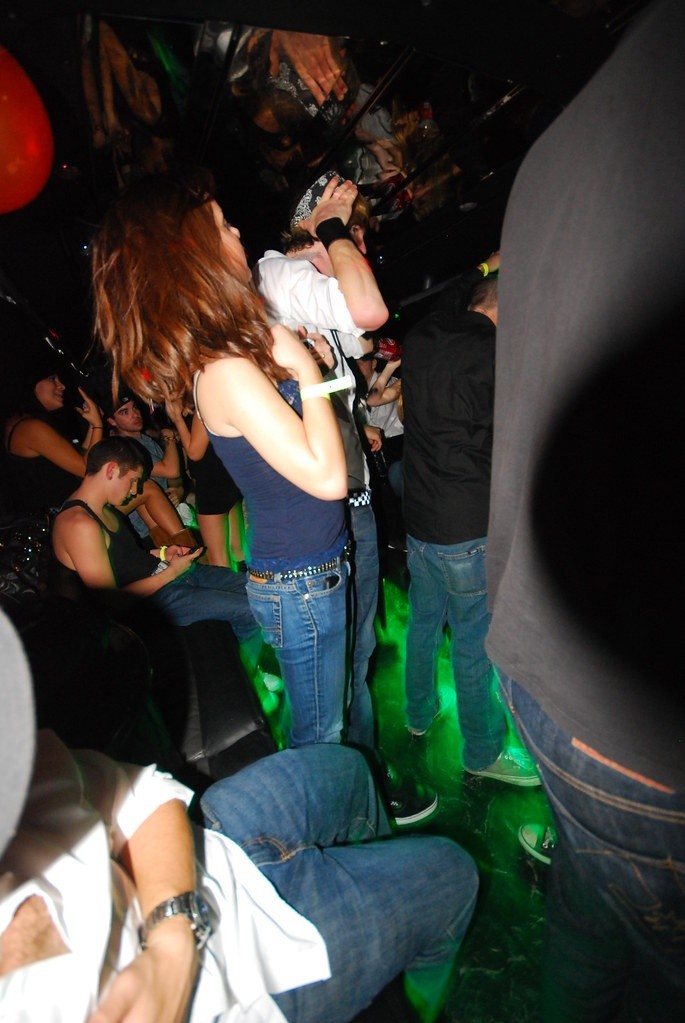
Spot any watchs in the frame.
[137,891,212,952]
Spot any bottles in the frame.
[367,334,403,361]
[1,516,51,614]
[416,102,440,147]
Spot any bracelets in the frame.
[92,427,103,429]
[480,262,488,277]
[300,375,352,401]
[315,216,354,251]
[167,437,177,442]
[160,546,166,561]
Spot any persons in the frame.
[80,1,421,199]
[0,168,479,1023]
[402,247,542,787]
[492,0,685,1023]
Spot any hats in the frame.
[100,389,135,420]
[373,337,403,361]
[290,170,347,235]
[270,61,343,124]
[0,609,38,869]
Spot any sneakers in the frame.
[405,998,459,1023]
[254,665,285,714]
[463,745,542,787]
[407,683,457,736]
[518,821,560,865]
[387,539,408,552]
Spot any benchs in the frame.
[1,560,268,788]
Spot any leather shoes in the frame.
[386,782,438,825]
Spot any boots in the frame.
[147,524,197,552]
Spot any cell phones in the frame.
[184,545,198,556]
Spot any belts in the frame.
[347,487,372,506]
[246,539,352,580]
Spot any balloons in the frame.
[0,48,52,215]
